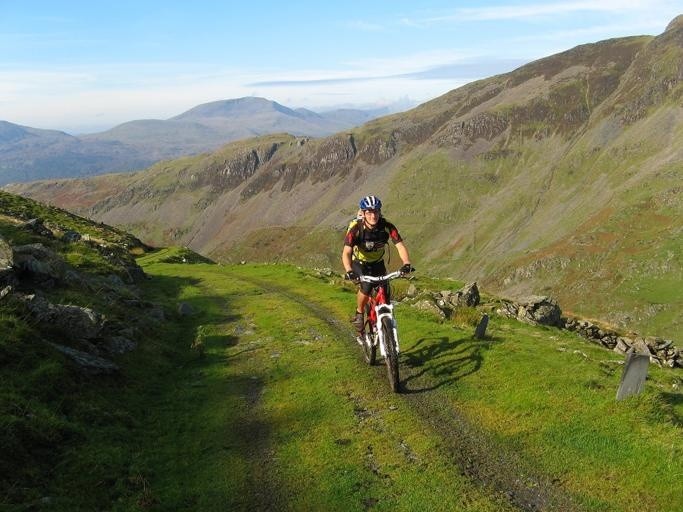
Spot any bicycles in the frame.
[341,266,415,391]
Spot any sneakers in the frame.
[353,306,365,332]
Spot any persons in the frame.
[341,195,415,334]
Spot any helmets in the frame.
[359,195,381,210]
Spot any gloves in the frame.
[400,263,412,275]
[347,270,359,283]
